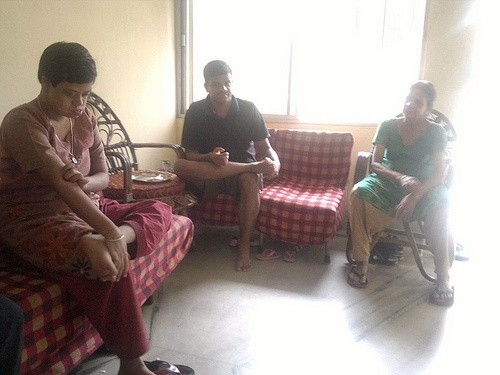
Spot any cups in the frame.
[161,160,171,172]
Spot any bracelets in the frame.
[401,175,408,180]
[105,235,125,242]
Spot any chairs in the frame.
[85,90,198,253]
[346,109,454,282]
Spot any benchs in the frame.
[0,129,353,374]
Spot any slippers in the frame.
[346,269,368,287]
[142,359,195,375]
[257,249,283,259]
[430,284,455,306]
[283,249,296,262]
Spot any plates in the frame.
[130,171,172,183]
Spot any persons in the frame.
[0,39,173,375]
[348,81,455,304]
[176,60,281,271]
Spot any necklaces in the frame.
[38,95,78,164]
